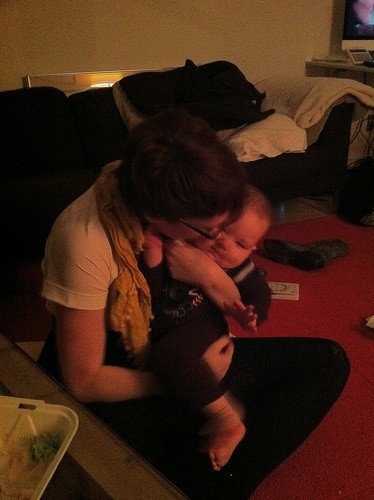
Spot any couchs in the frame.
[0,74,359,262]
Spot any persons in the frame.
[35,110,351,500]
[139,184,272,470]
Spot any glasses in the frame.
[177,217,223,239]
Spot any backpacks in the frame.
[338,157,374,226]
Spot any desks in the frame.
[307,60,374,84]
[0,331,189,500]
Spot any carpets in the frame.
[224,216,374,500]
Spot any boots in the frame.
[263,238,350,270]
